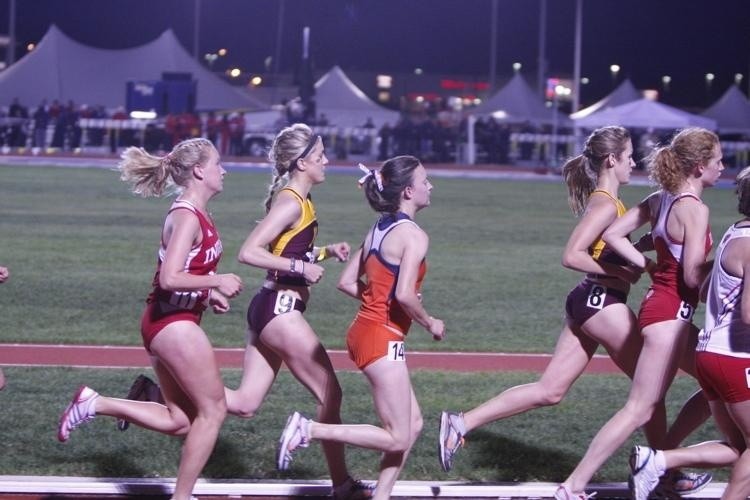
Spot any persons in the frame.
[58,138,244,500]
[1,97,572,165]
[276,154,446,499]
[0,265,9,392]
[628,167,750,500]
[438,125,714,494]
[554,129,725,499]
[119,123,375,500]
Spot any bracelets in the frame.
[320,246,330,260]
[300,260,304,276]
[290,257,296,276]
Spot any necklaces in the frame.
[186,194,213,216]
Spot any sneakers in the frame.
[553,483,598,500]
[116,372,158,432]
[647,468,713,500]
[57,383,100,443]
[627,444,661,500]
[437,409,466,474]
[328,475,380,500]
[275,409,313,473]
